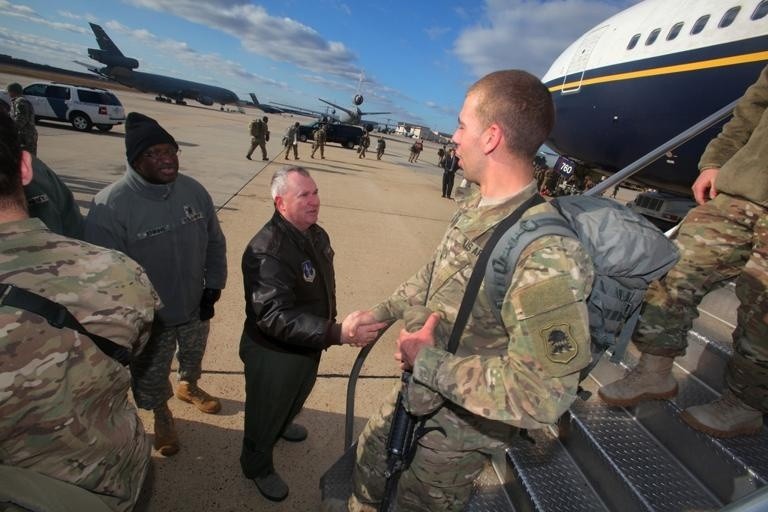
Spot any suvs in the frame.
[0,82,126,132]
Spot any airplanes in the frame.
[268,65,425,132]
[72,22,239,112]
[246,92,311,118]
[539,1,768,202]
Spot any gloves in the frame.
[199,289,221,321]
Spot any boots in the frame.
[598,354,678,406]
[680,385,764,438]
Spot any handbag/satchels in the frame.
[484,193,680,383]
[357,148,362,153]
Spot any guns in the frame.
[380,368,434,510]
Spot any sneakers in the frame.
[176,379,221,414]
[281,423,307,442]
[254,469,289,501]
[153,403,179,456]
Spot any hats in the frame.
[125,111,178,164]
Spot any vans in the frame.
[297,121,364,149]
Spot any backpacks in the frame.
[249,119,262,139]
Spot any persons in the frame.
[284,122,300,160]
[357,132,370,159]
[85,111,228,458]
[5,82,38,157]
[245,116,269,161]
[610,184,619,199]
[438,146,447,168]
[0,98,165,511]
[440,150,460,199]
[347,69,596,512]
[238,164,388,502]
[23,151,84,237]
[376,136,386,160]
[408,140,417,163]
[310,125,328,159]
[596,65,768,437]
[415,140,423,164]
